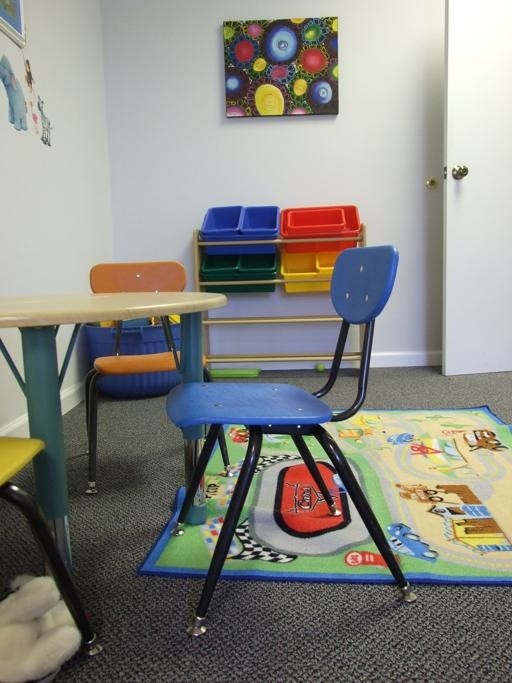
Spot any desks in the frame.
[0,291,228,580]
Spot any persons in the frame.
[21,52,40,120]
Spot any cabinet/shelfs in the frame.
[191,222,366,377]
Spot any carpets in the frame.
[137,403,511,588]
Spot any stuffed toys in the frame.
[0,573,82,683]
